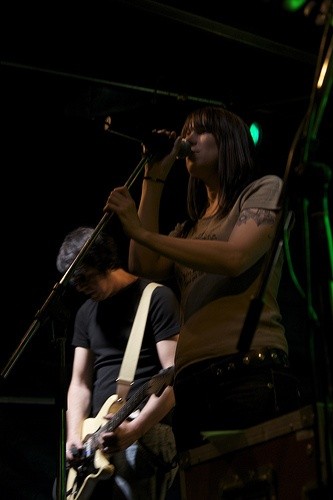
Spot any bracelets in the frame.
[143,177,168,182]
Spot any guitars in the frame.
[50,366,176,500]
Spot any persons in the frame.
[57,226,179,500]
[102,105,302,462]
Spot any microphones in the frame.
[93,116,191,159]
